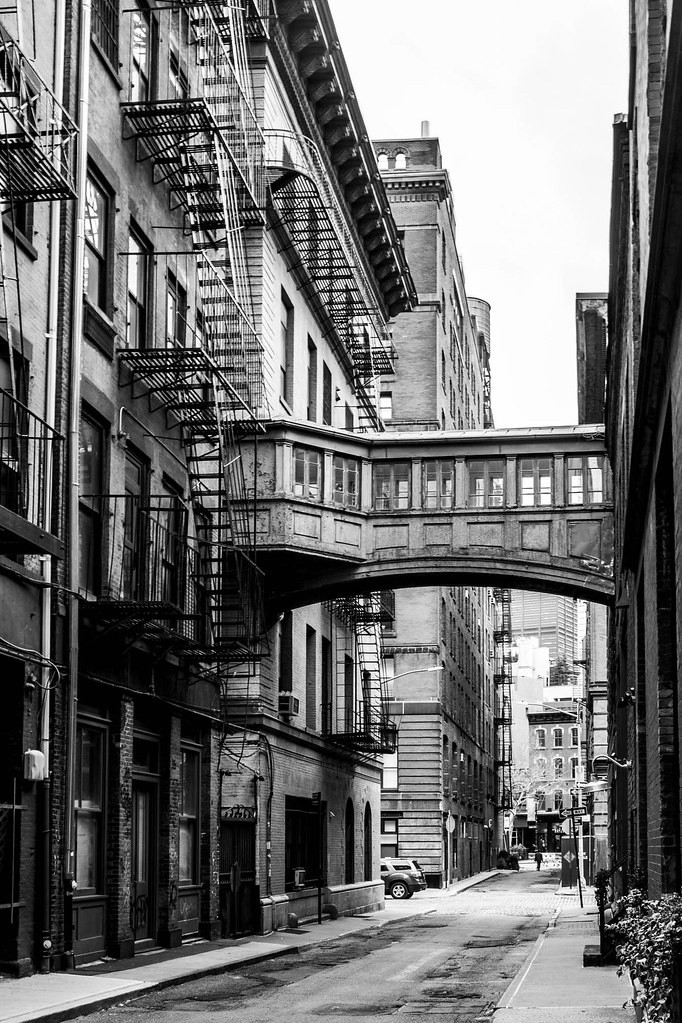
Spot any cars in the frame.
[381,857,428,899]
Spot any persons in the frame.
[534,849,543,871]
[531,839,561,853]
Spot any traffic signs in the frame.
[559,807,587,820]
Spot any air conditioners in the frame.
[488,589,492,597]
[278,696,300,717]
[294,868,305,887]
[491,597,495,605]
[460,753,464,762]
[490,650,494,657]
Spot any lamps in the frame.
[582,754,632,793]
